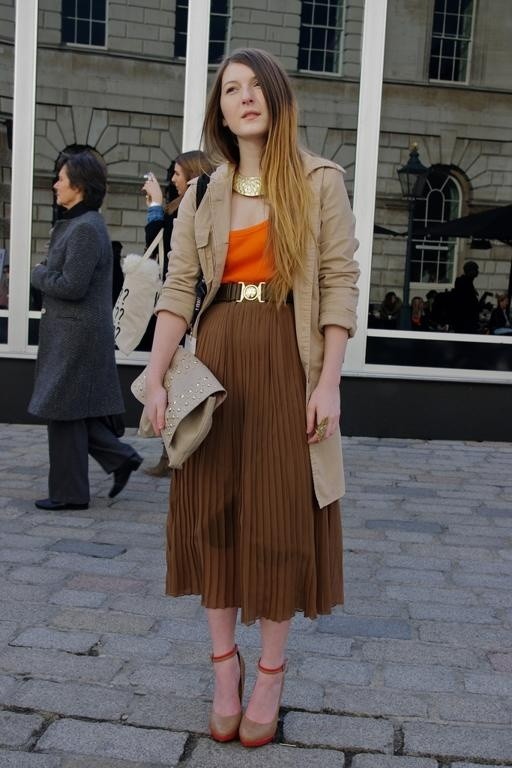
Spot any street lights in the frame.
[395,142,429,327]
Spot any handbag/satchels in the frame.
[112,226,165,356]
[130,344,228,472]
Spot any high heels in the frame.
[208,643,244,748]
[241,658,289,747]
[143,446,174,478]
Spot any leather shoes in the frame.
[35,497,89,511]
[107,452,144,500]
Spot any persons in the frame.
[370,262,512,333]
[137,151,214,475]
[31,147,143,511]
[138,50,359,747]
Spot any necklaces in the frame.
[231,170,263,197]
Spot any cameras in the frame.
[143,174,148,181]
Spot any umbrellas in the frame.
[374,224,401,236]
[402,204,512,310]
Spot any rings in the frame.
[314,418,328,443]
